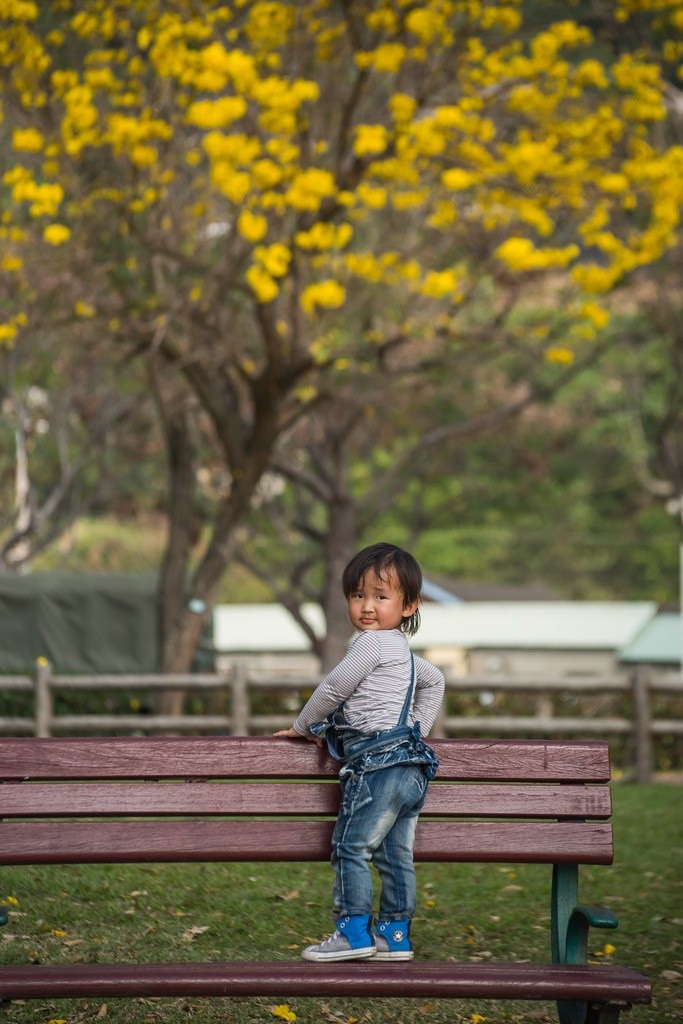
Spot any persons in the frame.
[270,544,445,962]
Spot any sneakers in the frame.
[300,913,377,962]
[357,918,414,962]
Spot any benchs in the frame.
[0,737,652,1024]
